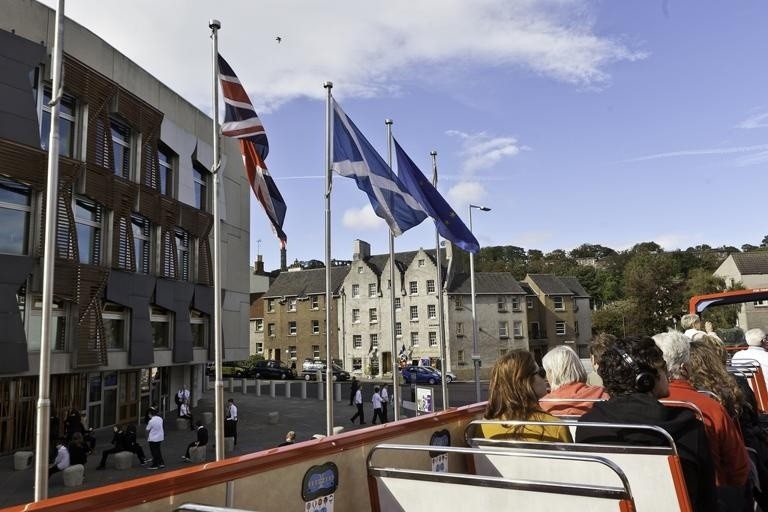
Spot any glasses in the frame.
[531,367,547,377]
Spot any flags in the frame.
[330,95,427,237]
[393,135,481,256]
[215,51,287,248]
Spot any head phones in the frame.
[612,342,656,394]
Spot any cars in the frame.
[208,358,350,381]
[395,365,457,385]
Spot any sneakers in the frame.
[145,466,159,470]
[145,457,152,462]
[159,464,165,469]
[141,463,148,466]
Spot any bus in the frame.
[2,288,768,510]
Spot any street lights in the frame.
[469,204,492,401]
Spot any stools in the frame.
[176,418,190,430]
[269,411,279,424]
[190,445,206,465]
[333,426,344,435]
[201,412,213,424]
[14,451,33,470]
[114,451,133,471]
[224,437,234,452]
[63,464,85,487]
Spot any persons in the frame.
[279,431,297,447]
[48,384,209,476]
[350,375,389,427]
[226,399,238,444]
[482,313,768,511]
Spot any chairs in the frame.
[717,327,743,346]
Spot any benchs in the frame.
[463,420,693,512]
[366,444,636,512]
[726,358,768,413]
[173,502,255,512]
[538,398,710,443]
[697,390,722,405]
